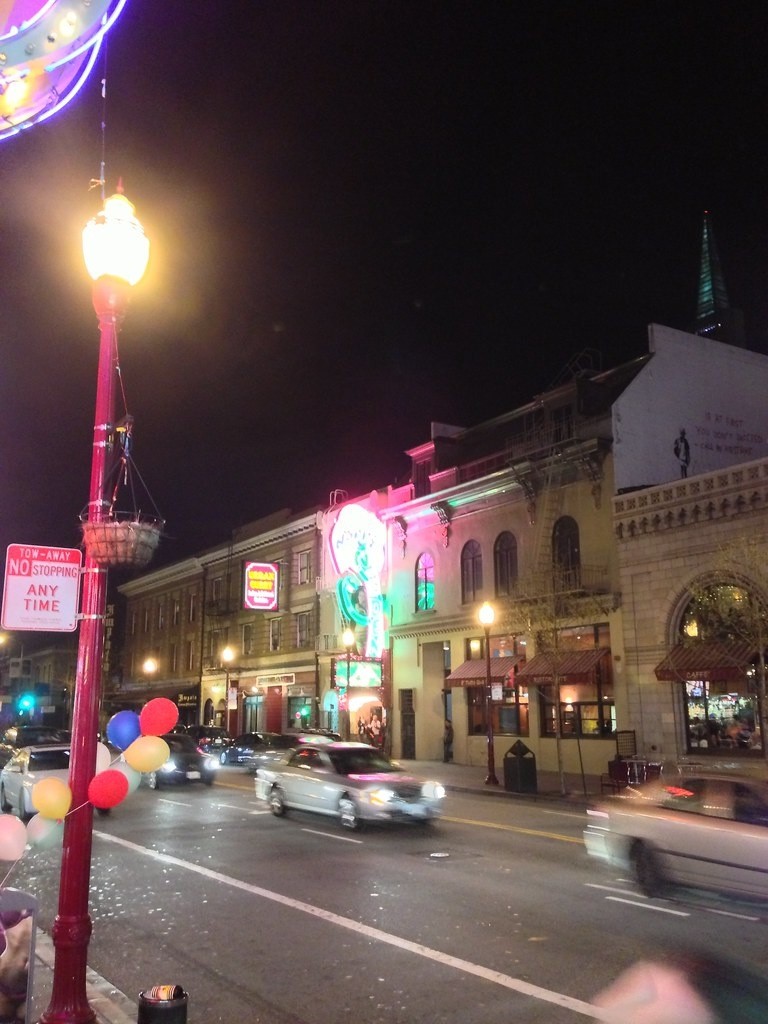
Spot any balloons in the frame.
[0,697,179,861]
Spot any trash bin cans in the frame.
[502,739,537,793]
[137,990,189,1024]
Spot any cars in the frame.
[120,730,218,792]
[254,740,448,832]
[0,725,70,771]
[0,744,72,822]
[582,767,768,904]
[171,721,342,772]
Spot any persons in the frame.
[443,718,454,763]
[593,719,612,736]
[209,717,214,725]
[357,714,386,746]
[220,713,226,726]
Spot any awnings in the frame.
[445,656,523,689]
[653,640,759,682]
[513,648,609,687]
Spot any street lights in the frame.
[476,600,501,786]
[220,644,235,733]
[143,658,157,702]
[0,632,25,726]
[25,175,156,1024]
